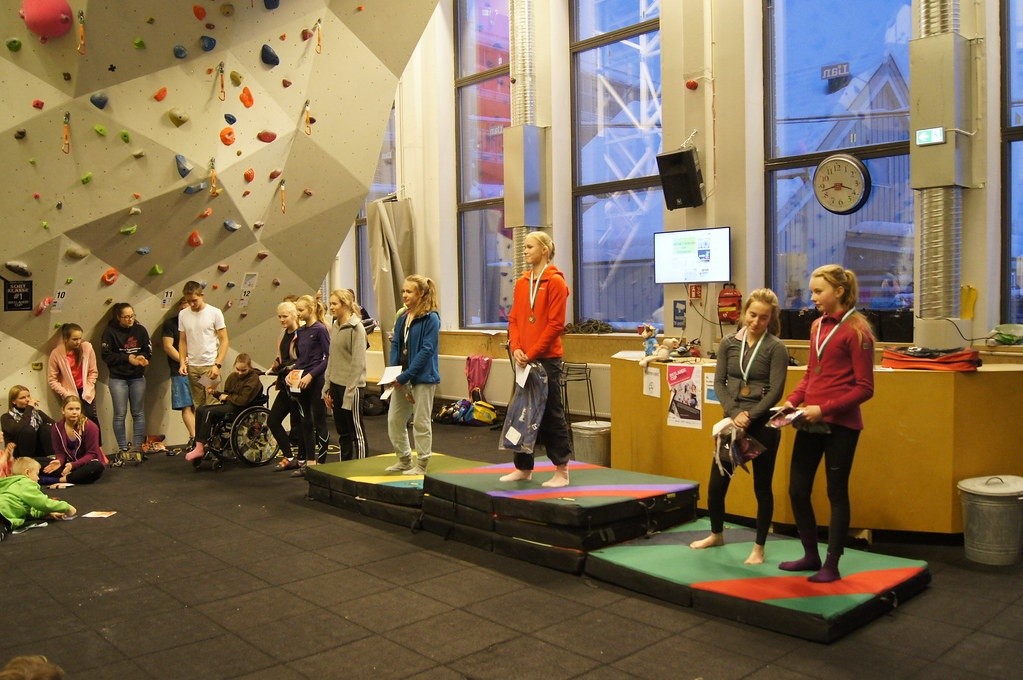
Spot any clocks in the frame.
[812,154,871,215]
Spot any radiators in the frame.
[365,350,612,416]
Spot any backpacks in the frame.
[435,399,498,426]
[882,346,983,372]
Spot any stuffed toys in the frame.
[640,325,678,367]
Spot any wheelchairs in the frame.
[185,367,291,467]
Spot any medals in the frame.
[815,366,822,375]
[403,349,407,355]
[529,316,535,323]
[741,386,750,396]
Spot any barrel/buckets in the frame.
[957,474,1023,565]
[571,421,611,464]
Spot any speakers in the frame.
[656,147,707,211]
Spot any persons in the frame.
[0,431,76,544]
[499,230,570,489]
[690,286,787,564]
[1,385,55,458]
[178,281,229,408]
[385,275,441,476]
[38,395,104,486]
[681,384,698,408]
[101,303,152,463]
[185,353,264,461]
[48,323,110,463]
[778,265,875,582]
[266,289,375,477]
[161,296,197,452]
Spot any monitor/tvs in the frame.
[653,226,732,283]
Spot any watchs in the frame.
[215,363,222,368]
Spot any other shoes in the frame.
[186,438,194,452]
[272,458,298,471]
[291,469,305,476]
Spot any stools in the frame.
[558,362,596,427]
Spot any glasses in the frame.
[118,314,136,320]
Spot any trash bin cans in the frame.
[571,420,611,468]
[955,471,1021,568]
